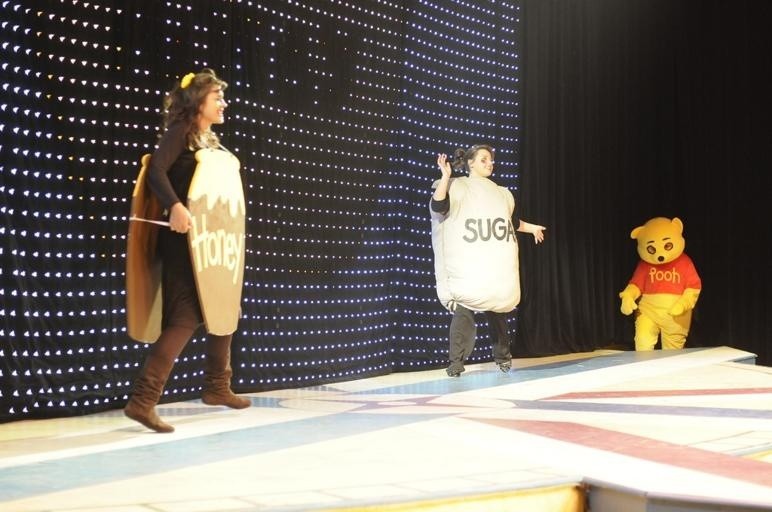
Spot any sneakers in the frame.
[500,355,511,373]
[445,367,465,378]
[124,398,174,432]
[201,390,250,409]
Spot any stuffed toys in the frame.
[618,216,703,352]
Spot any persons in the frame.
[427,144,548,378]
[124,68,251,432]
